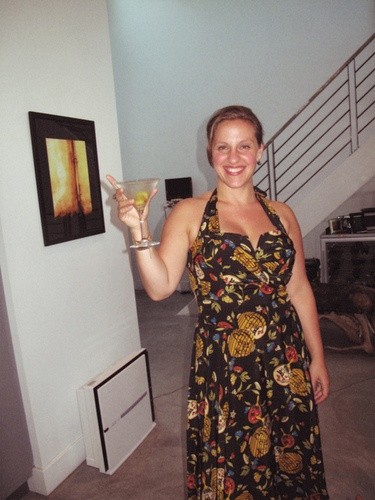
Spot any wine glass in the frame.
[115,179,160,249]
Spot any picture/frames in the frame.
[28,111,105,247]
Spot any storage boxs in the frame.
[320,230,375,287]
